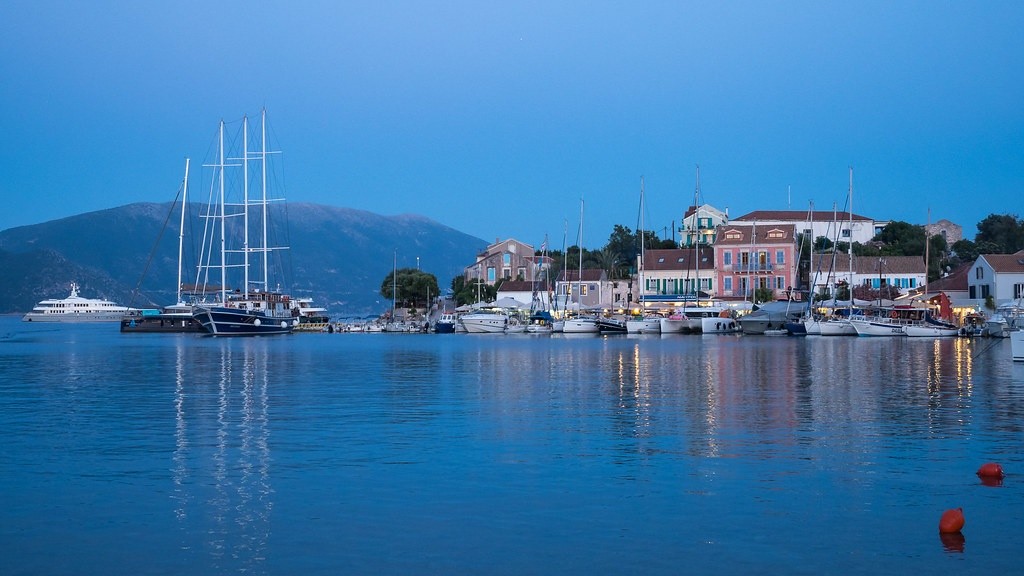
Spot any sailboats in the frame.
[849,246,908,337]
[331,165,893,336]
[902,207,959,337]
[118,158,208,334]
[191,107,300,335]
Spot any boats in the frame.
[22,282,137,322]
[291,298,332,332]
[957,294,1024,363]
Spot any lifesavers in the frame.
[892,311,897,317]
[540,320,544,325]
